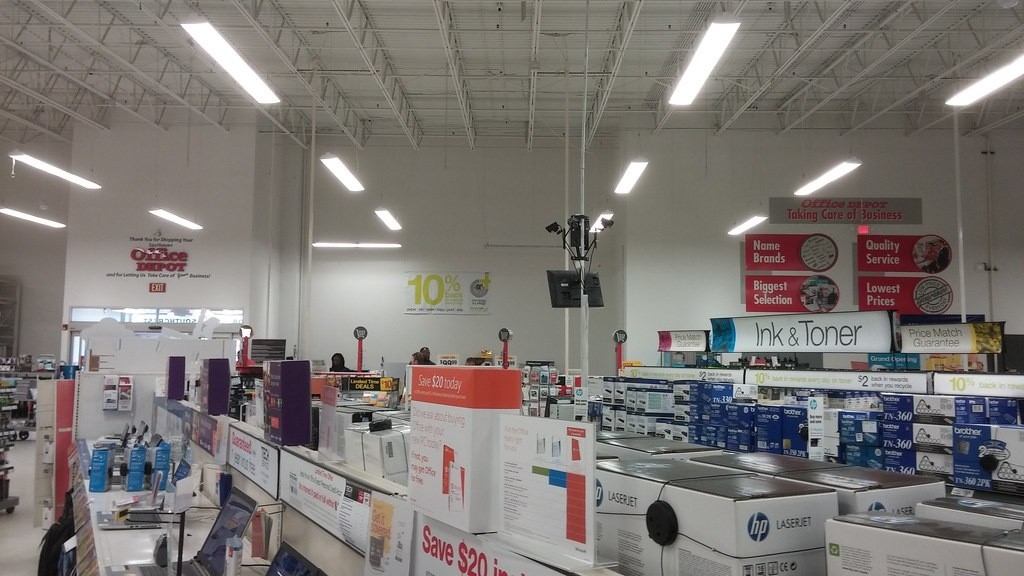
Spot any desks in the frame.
[66,437,280,576]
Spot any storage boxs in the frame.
[166,356,185,400]
[102,375,133,411]
[119,447,146,491]
[89,446,117,492]
[602,377,1023,500]
[397,364,520,532]
[145,441,172,491]
[170,458,192,485]
[197,358,231,415]
[263,358,310,446]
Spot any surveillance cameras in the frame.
[601,218,614,227]
[545,222,559,233]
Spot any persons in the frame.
[411,352,425,365]
[329,353,350,372]
[401,348,436,404]
[236,349,255,366]
[924,240,949,273]
[465,358,476,366]
[802,284,838,312]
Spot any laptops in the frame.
[95,418,328,576]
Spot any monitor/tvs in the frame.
[546,270,605,308]
